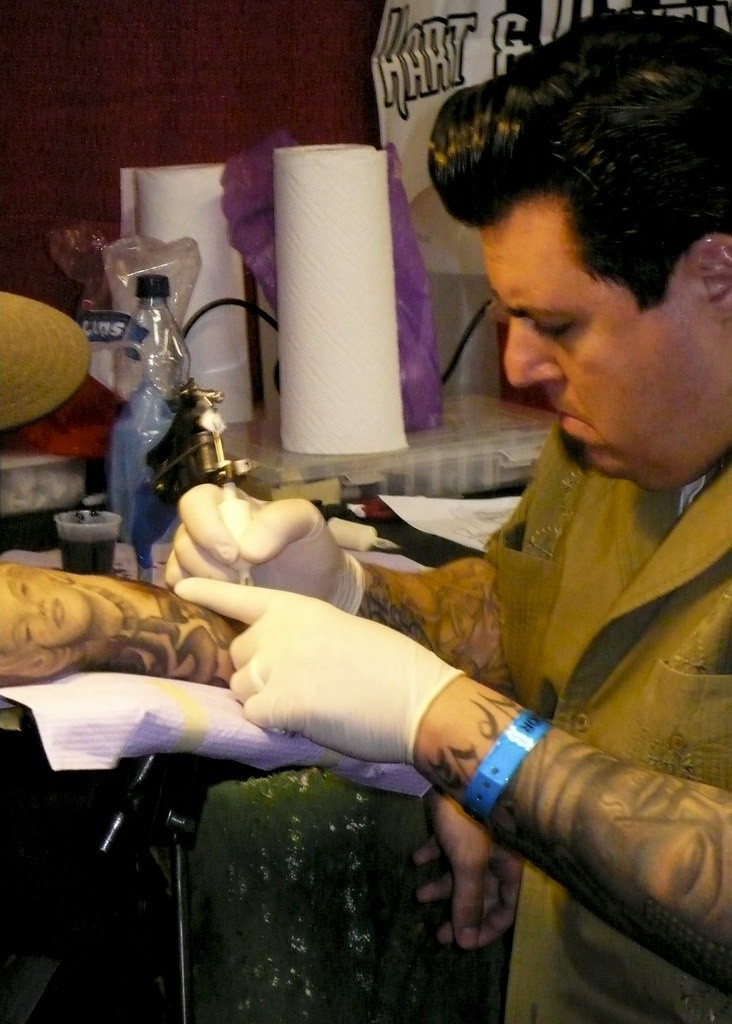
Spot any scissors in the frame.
[322,498,395,518]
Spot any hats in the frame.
[0,291,92,429]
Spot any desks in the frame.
[220,393,559,506]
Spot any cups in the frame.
[54,510,123,576]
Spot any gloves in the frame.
[165,483,366,615]
[174,578,466,764]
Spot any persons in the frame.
[166,9,732,1024]
[0,566,522,947]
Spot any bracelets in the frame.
[461,709,553,829]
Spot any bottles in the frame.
[114,274,190,423]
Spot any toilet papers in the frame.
[121,164,253,425]
[275,145,407,454]
